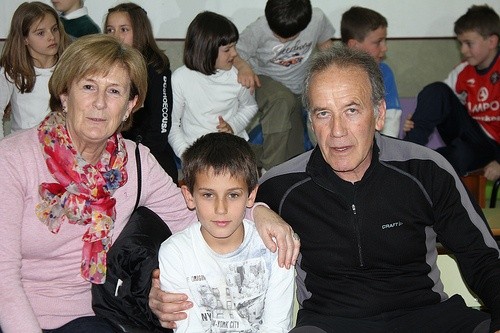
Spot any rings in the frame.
[291,233,301,241]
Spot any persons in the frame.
[49,0,101,38]
[340,6,402,138]
[0,1,74,141]
[232,0,336,178]
[167,11,259,160]
[149,44,500,333]
[401,3,500,181]
[157,132,297,333]
[0,34,301,333]
[104,2,181,187]
